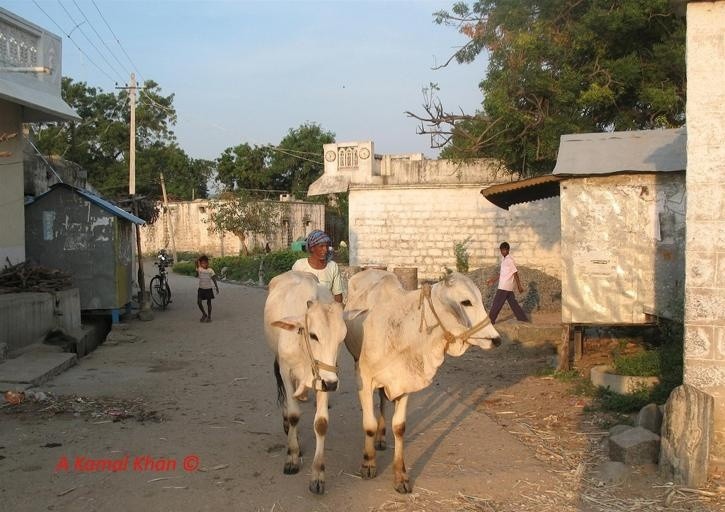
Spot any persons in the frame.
[194,255,219,324]
[290,228,344,409]
[485,242,529,325]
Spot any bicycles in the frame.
[149,248,174,313]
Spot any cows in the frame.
[261,266,369,493]
[343,267,504,496]
